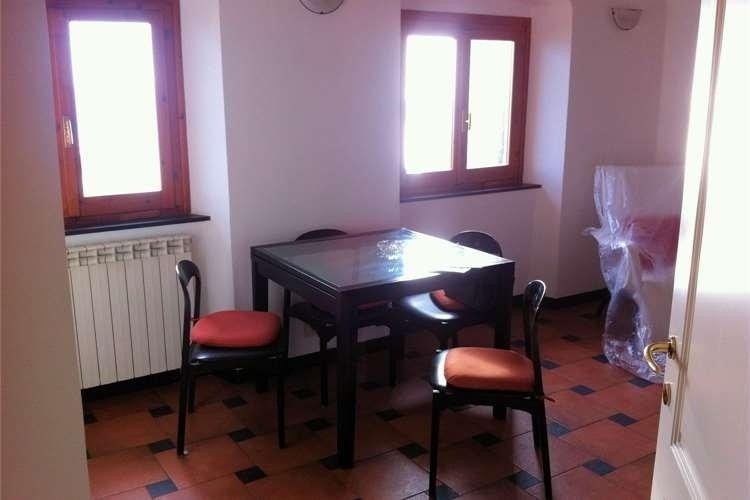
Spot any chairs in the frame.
[393,227,503,378]
[175,258,288,459]
[429,279,552,499]
[597,163,683,364]
[282,228,398,409]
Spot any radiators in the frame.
[66,233,196,390]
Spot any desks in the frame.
[250,228,516,471]
